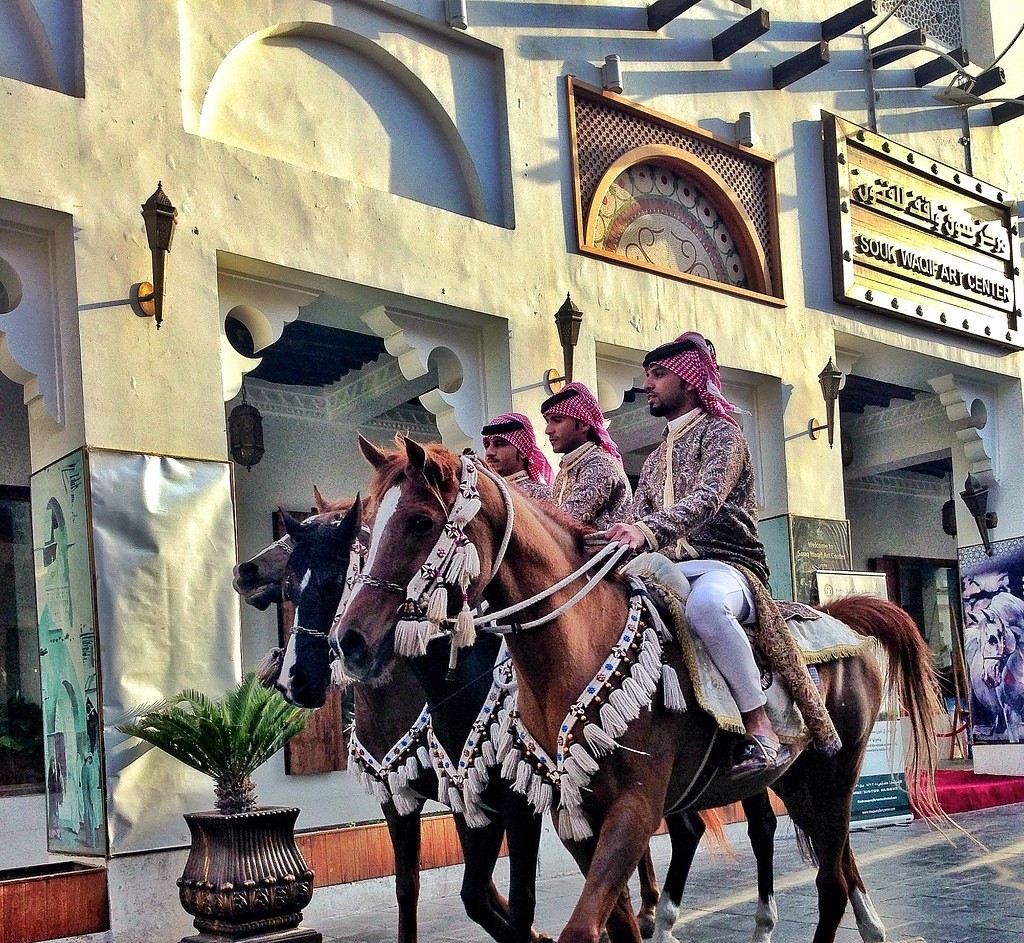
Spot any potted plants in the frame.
[115,671,320,943]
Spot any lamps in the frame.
[810,356,842,449]
[941,457,957,540]
[137,180,179,330]
[958,471,998,557]
[547,291,584,394]
[227,374,266,473]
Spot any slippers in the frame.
[731,735,791,780]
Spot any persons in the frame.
[603,332,844,781]
[481,413,555,502]
[539,382,634,532]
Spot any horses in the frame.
[233,432,947,943]
